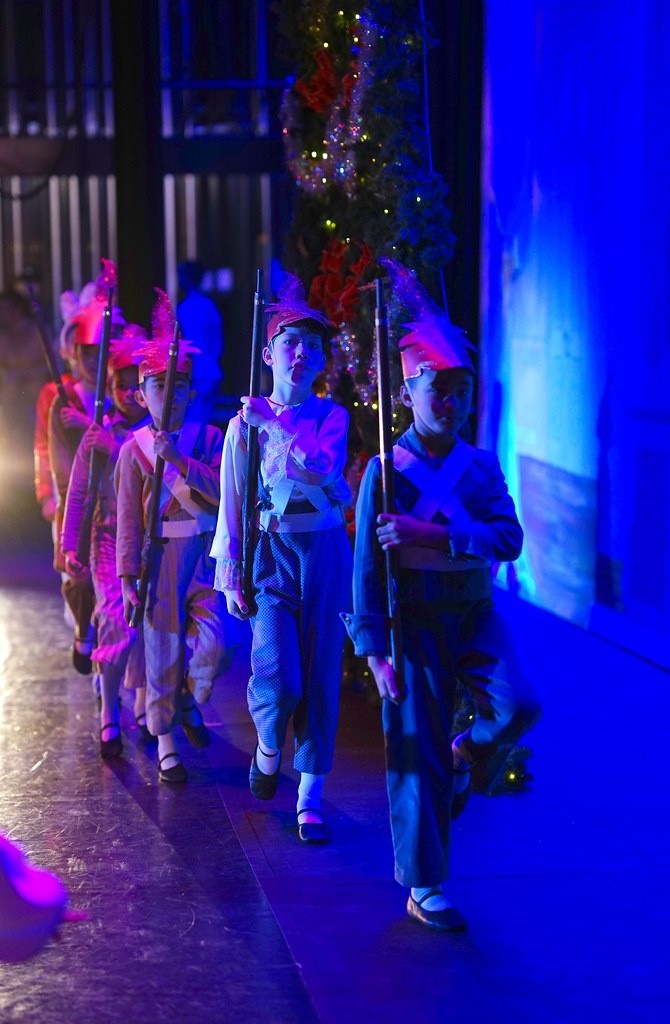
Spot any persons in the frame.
[32,315,155,760]
[208,304,353,844]
[338,329,526,932]
[114,350,226,784]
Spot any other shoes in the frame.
[98,720,123,758]
[156,751,187,784]
[130,703,154,738]
[404,889,465,935]
[451,763,471,820]
[180,693,211,748]
[296,807,331,845]
[70,619,97,675]
[249,741,282,801]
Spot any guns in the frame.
[129,319,182,627]
[28,287,76,459]
[74,287,114,565]
[241,268,264,612]
[373,278,407,697]
